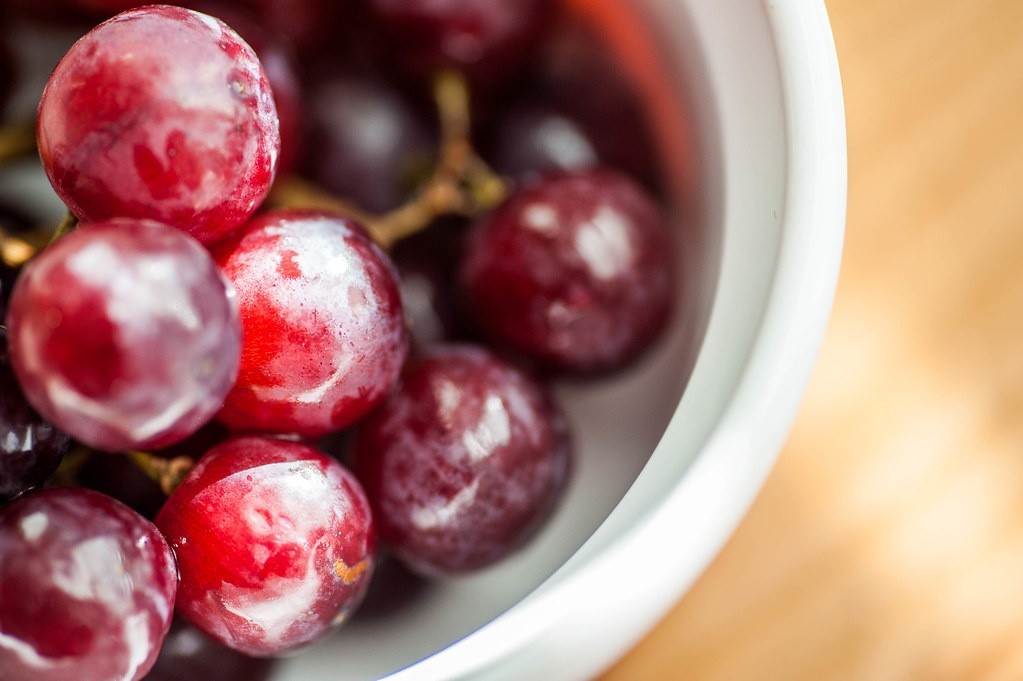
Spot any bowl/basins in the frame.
[1,0,848,681]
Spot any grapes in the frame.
[0,0,680,680]
